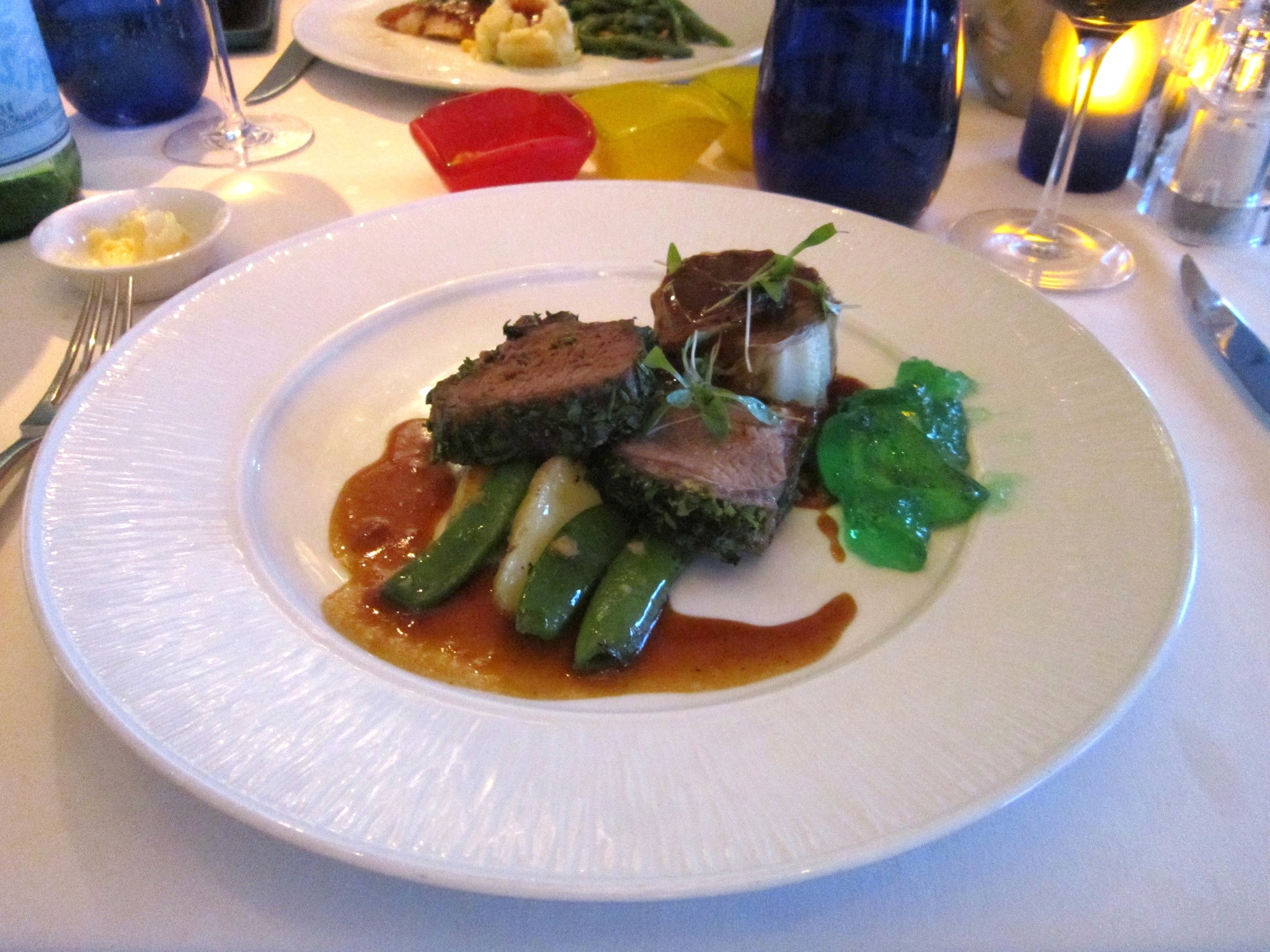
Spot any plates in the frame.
[21,178,1196,909]
[291,1,777,91]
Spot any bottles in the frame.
[0,0,83,243]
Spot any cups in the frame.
[752,0,961,225]
[31,0,211,127]
[962,0,1055,119]
[1125,1,1270,251]
[1017,9,1167,194]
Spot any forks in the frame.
[0,273,134,476]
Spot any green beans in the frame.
[559,0,731,60]
[376,458,685,672]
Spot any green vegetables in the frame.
[639,223,845,436]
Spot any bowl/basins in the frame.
[407,87,598,196]
[565,79,729,175]
[30,185,234,301]
[687,67,762,167]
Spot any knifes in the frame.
[1179,254,1270,416]
[241,38,314,102]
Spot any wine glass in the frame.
[163,0,314,168]
[949,0,1198,290]
